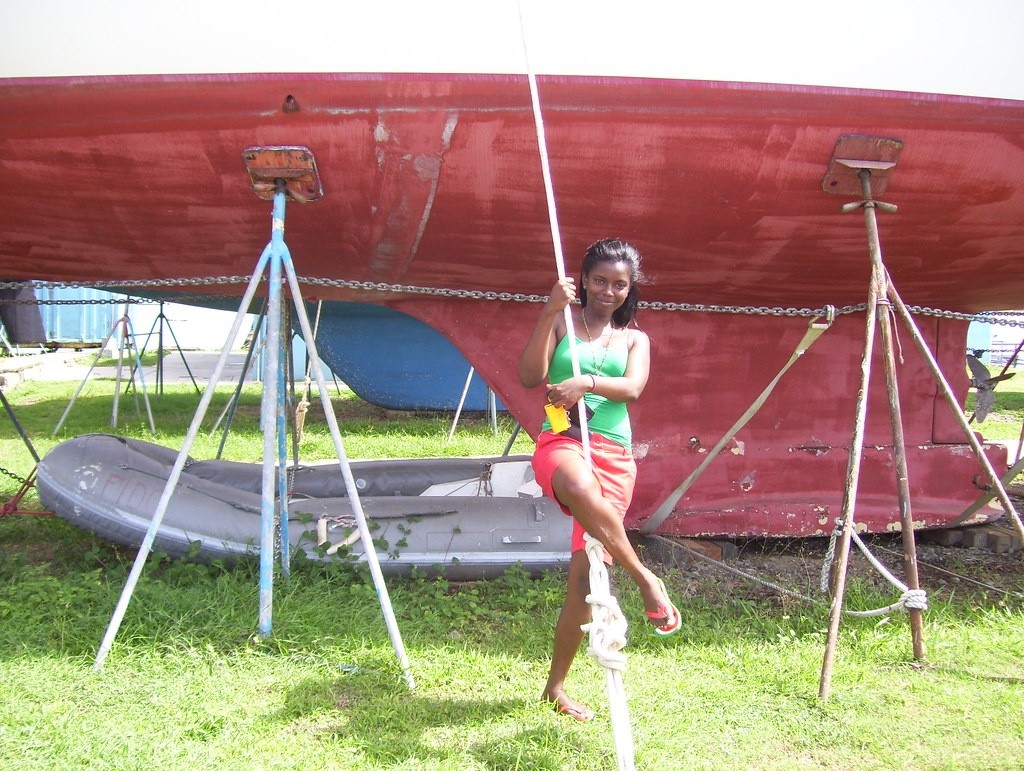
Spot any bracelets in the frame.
[587,373,596,391]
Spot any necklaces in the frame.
[581,307,615,378]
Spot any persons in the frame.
[517,240,682,723]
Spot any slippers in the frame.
[643,577,682,637]
[557,706,594,723]
